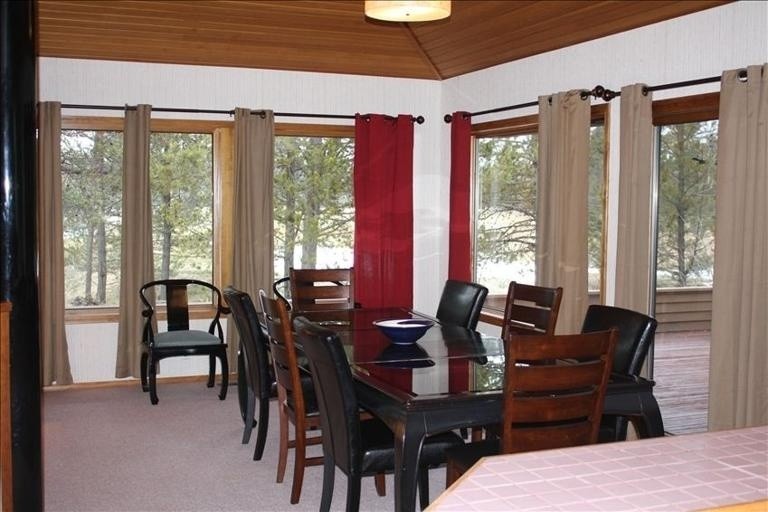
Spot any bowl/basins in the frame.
[374,344,435,367]
[372,318,436,345]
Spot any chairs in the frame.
[436,279,488,332]
[293,316,468,512]
[289,266,355,312]
[447,326,617,487]
[272,277,361,312]
[222,285,316,463]
[581,304,659,442]
[501,281,563,341]
[139,279,232,405]
[259,290,386,504]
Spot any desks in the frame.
[421,423,768,512]
[237,305,662,512]
